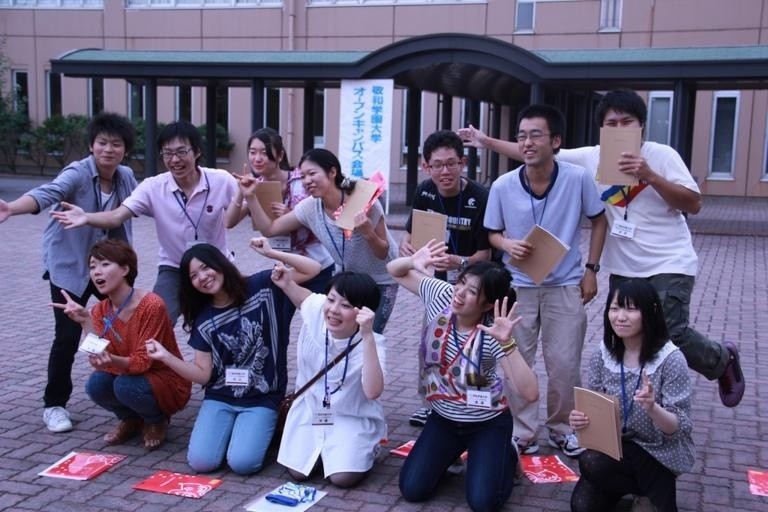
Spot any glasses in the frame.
[160,147,193,158]
[513,130,551,142]
[427,158,462,171]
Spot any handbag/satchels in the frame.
[274,395,294,432]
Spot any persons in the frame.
[397,127,502,428]
[480,100,610,457]
[457,85,747,408]
[267,259,392,489]
[45,233,191,451]
[385,238,543,510]
[142,233,324,479]
[565,276,699,512]
[223,124,339,399]
[45,117,241,331]
[0,110,157,435]
[237,145,404,336]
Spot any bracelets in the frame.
[503,337,517,356]
[231,198,243,210]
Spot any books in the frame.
[409,209,447,256]
[250,180,285,233]
[508,224,573,286]
[334,177,380,232]
[571,384,626,465]
[596,123,643,185]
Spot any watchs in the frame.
[583,262,602,274]
[460,256,471,269]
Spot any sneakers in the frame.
[548,431,586,457]
[409,407,432,426]
[42,406,73,432]
[717,341,745,407]
[510,435,539,455]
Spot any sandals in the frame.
[143,415,171,451]
[104,418,137,446]
